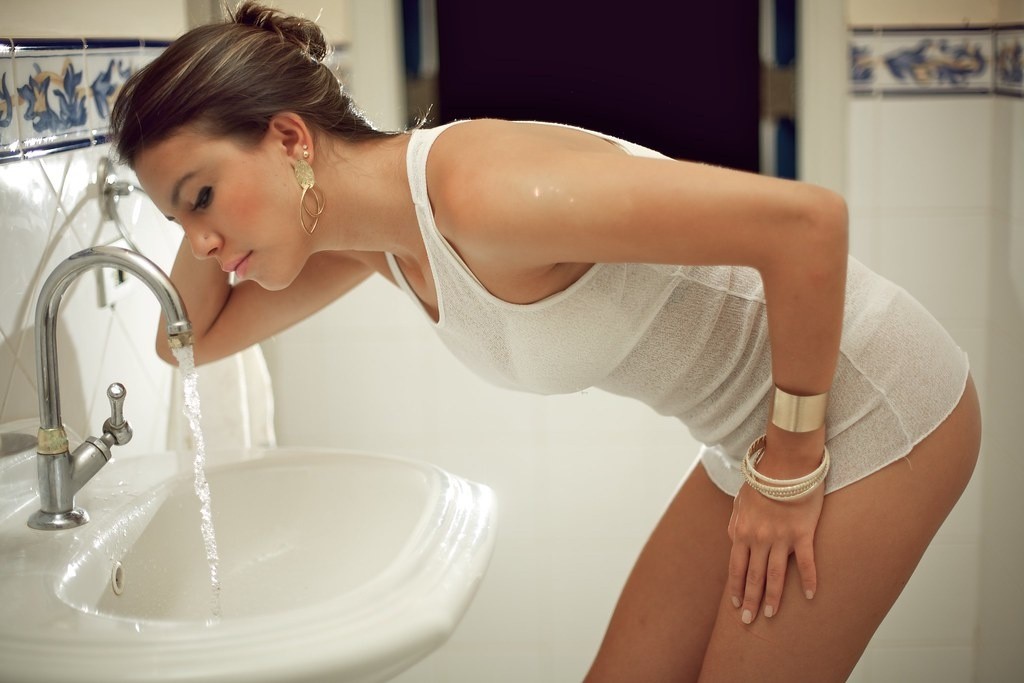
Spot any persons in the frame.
[110,0,980,683]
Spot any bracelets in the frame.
[772,388,828,432]
[741,435,829,500]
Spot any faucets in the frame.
[25,247,195,513]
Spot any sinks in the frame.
[2,417,87,521]
[1,446,501,681]
